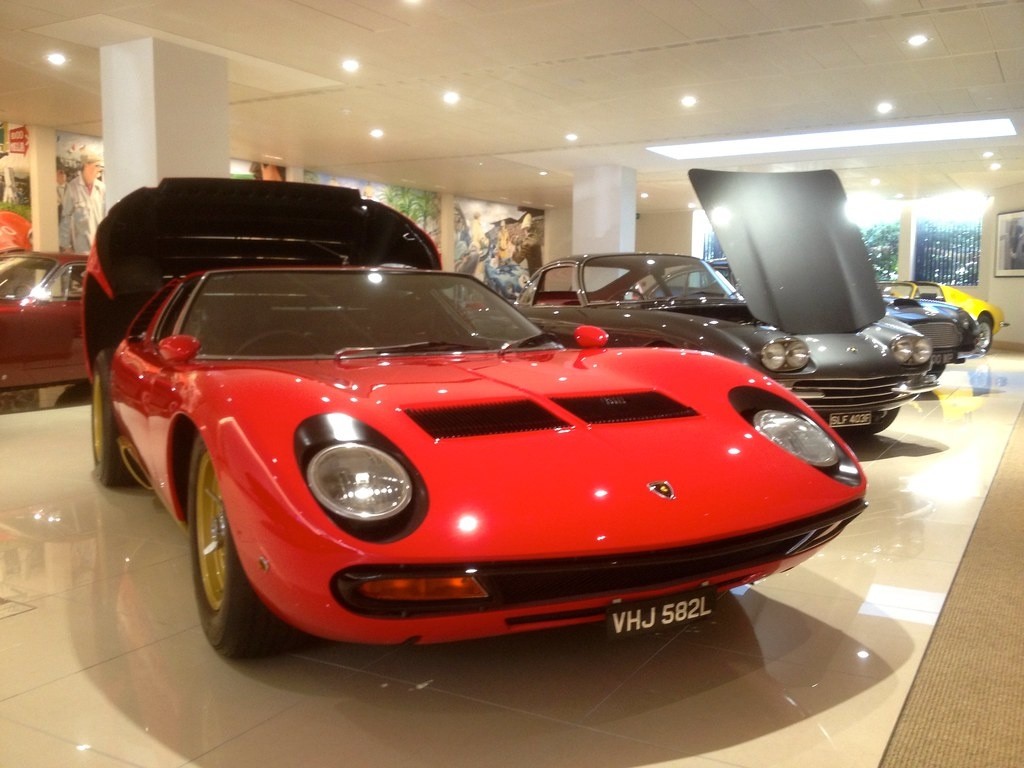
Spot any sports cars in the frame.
[876,278,1011,345]
[638,255,981,381]
[506,168,938,436]
[81,175,868,664]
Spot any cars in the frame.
[0,250,103,393]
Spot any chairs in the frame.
[918,292,938,300]
[385,297,459,346]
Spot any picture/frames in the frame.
[994,210,1024,278]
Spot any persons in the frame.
[56,148,106,294]
